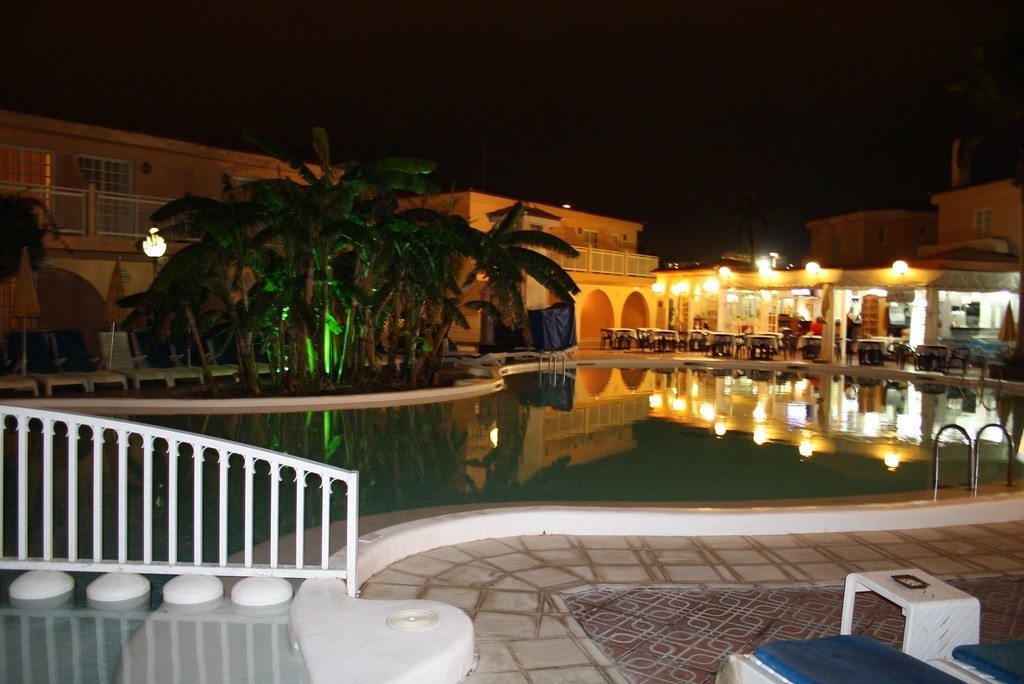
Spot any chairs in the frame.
[0,328,281,395]
[600,329,971,374]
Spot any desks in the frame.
[637,327,662,349]
[746,336,775,360]
[709,332,737,357]
[605,328,636,349]
[802,335,822,358]
[650,330,677,352]
[757,332,784,354]
[873,336,902,360]
[915,345,948,372]
[857,338,888,366]
[679,330,711,351]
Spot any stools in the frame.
[839,567,983,661]
[717,634,1024,684]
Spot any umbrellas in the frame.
[816,284,831,326]
[12,244,130,377]
[998,299,1018,353]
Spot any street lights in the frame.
[142,227,167,279]
